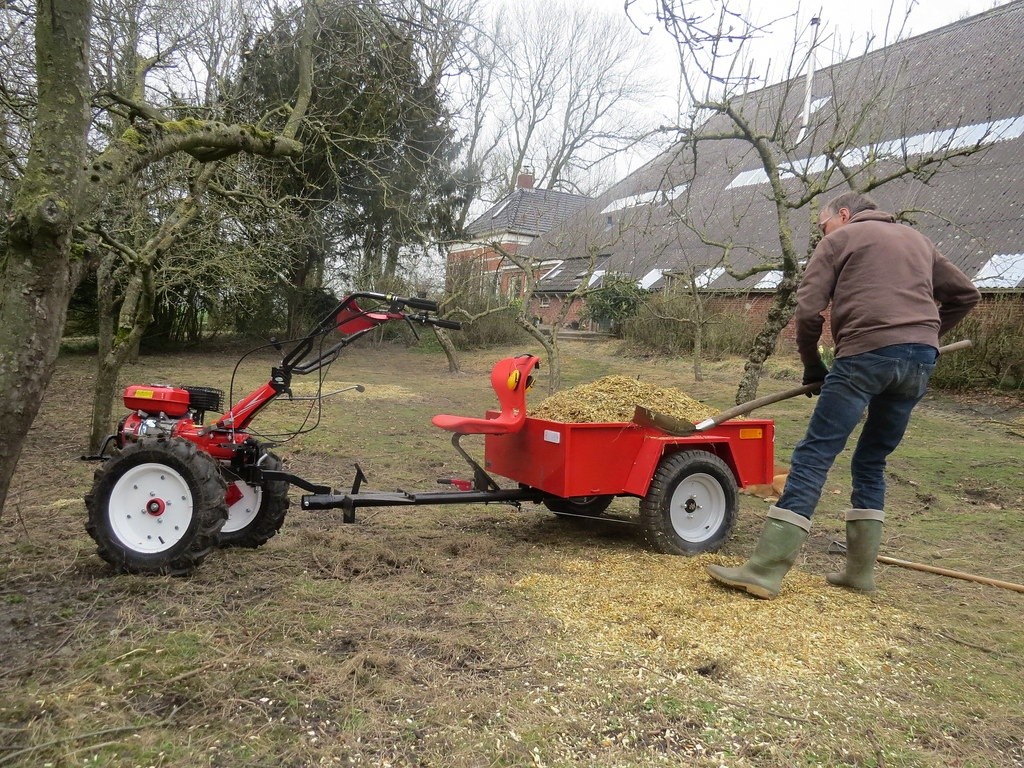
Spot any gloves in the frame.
[802,363,829,398]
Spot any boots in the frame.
[706,505,812,600]
[826,509,884,597]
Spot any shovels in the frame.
[635,339,971,438]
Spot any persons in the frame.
[702,187,982,599]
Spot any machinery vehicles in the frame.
[80,292,777,581]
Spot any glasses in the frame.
[818,216,832,230]
